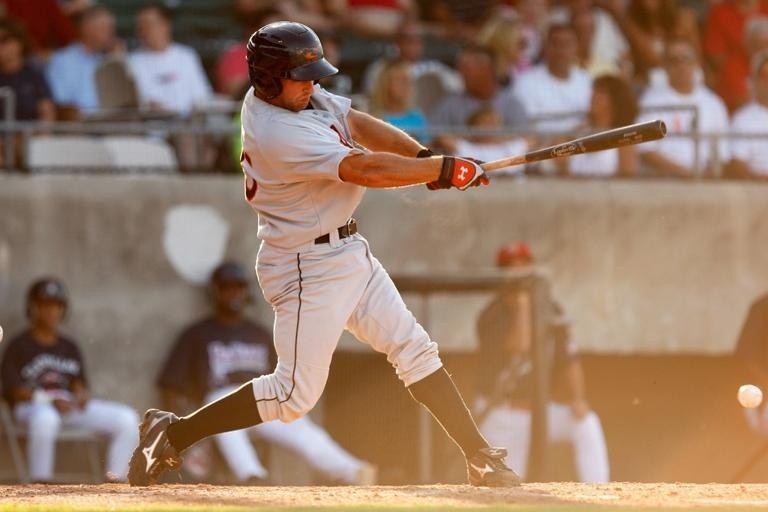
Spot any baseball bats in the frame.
[428,119,667,189]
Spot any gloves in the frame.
[440,155,489,190]
[417,150,453,189]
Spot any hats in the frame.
[496,247,530,266]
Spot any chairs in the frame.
[0,395,106,484]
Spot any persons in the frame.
[1,0,767,180]
[469,241,610,483]
[128,22,522,488]
[1,276,140,484]
[157,260,377,487]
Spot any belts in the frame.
[314,218,357,244]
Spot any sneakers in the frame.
[466,447,520,486]
[127,408,180,487]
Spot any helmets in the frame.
[212,263,252,315]
[26,280,67,322]
[246,22,338,101]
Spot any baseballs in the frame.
[737,385,764,407]
